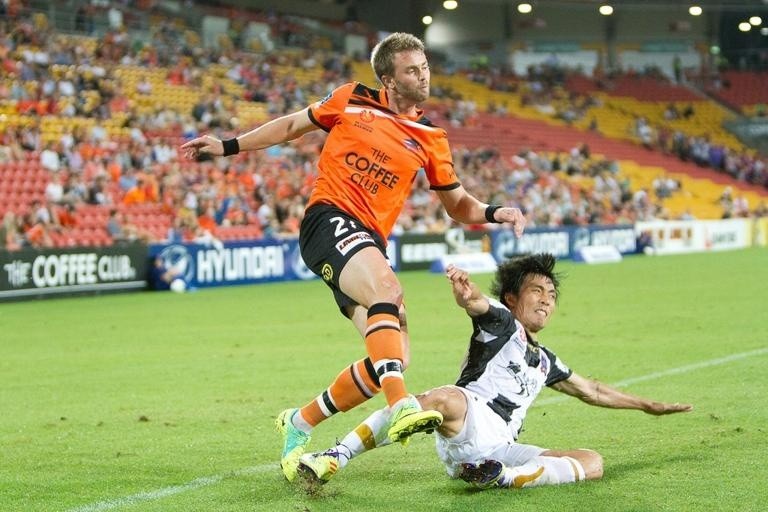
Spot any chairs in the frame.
[1,0,768,252]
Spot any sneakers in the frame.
[296,452,340,486]
[388,407,444,442]
[459,459,506,490]
[275,408,311,483]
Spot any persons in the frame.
[148,254,200,291]
[298,253,693,489]
[180,32,527,482]
